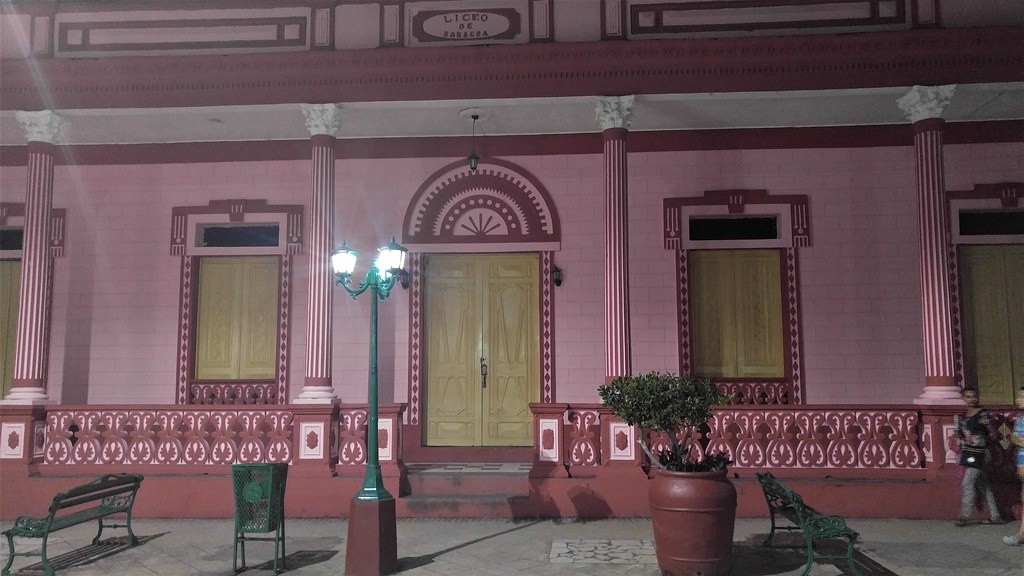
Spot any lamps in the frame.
[550,264,562,287]
[466,113,480,172]
[398,268,409,289]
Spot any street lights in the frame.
[331,235,411,576]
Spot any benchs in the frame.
[0,472,145,576]
[756,471,860,576]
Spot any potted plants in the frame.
[597,368,739,576]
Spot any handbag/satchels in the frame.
[958,446,986,468]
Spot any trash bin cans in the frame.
[231,463,290,576]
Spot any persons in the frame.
[998,386,1024,546]
[951,388,1004,526]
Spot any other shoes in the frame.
[1003,535,1021,545]
[953,520,971,526]
[983,517,1003,524]
